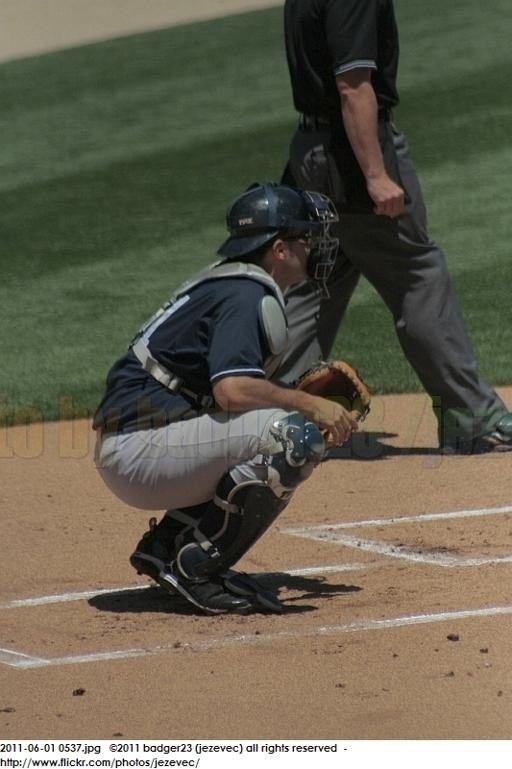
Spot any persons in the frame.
[85,178,367,621]
[281,0,512,453]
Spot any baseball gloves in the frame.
[293,359,371,451]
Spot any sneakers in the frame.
[439,413,512,454]
[130,519,252,614]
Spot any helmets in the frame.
[216,182,339,300]
